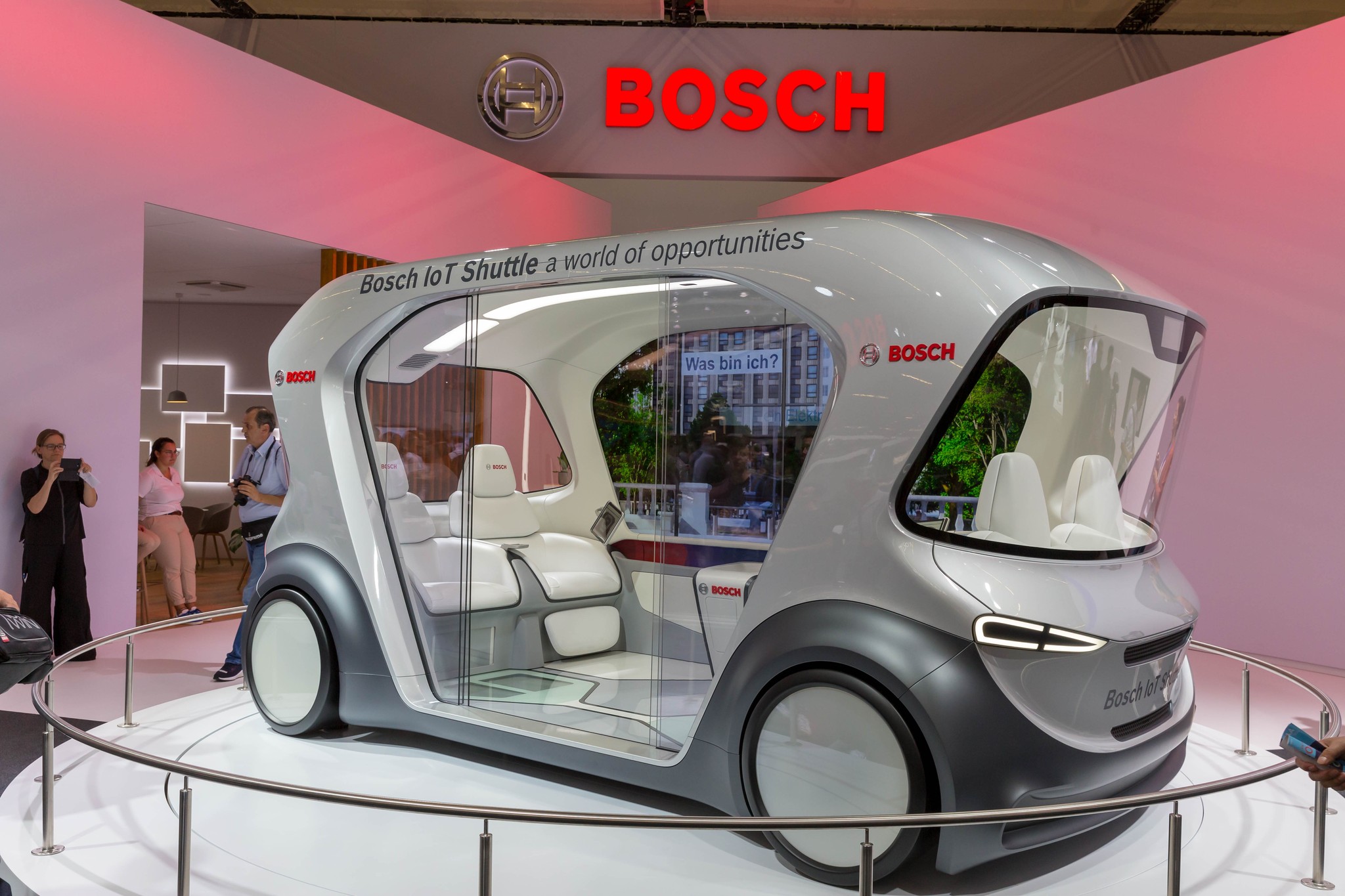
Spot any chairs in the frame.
[153,503,235,572]
[966,452,1150,553]
[375,441,623,616]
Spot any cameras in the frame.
[233,475,257,506]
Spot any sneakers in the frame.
[175,606,213,625]
[213,662,244,682]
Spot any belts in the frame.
[165,510,182,515]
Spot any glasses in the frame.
[43,444,66,450]
[161,451,180,456]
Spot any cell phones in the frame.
[60,457,82,470]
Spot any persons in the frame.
[662,428,806,505]
[1294,735,1345,792]
[140,437,213,625]
[373,414,477,486]
[212,405,290,682]
[18,428,99,662]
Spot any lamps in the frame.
[166,293,189,402]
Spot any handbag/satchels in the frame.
[0,607,54,694]
[240,515,277,546]
